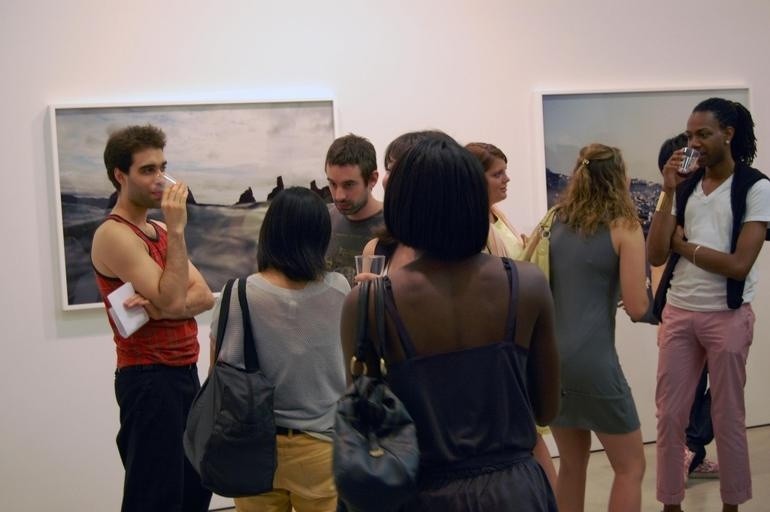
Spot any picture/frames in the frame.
[48,98,344,313]
[535,81,754,232]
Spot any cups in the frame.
[154,173,177,191]
[677,147,701,176]
[355,253,386,276]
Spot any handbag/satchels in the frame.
[333,377,419,510]
[183,361,276,497]
[518,205,559,282]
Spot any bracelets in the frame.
[691,244,703,265]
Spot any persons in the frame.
[518,139,658,511]
[638,98,770,512]
[314,132,391,285]
[87,125,216,512]
[357,128,452,278]
[460,142,557,511]
[339,137,564,512]
[645,130,727,490]
[208,185,356,511]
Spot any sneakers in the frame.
[690,461,721,478]
[685,446,695,486]
